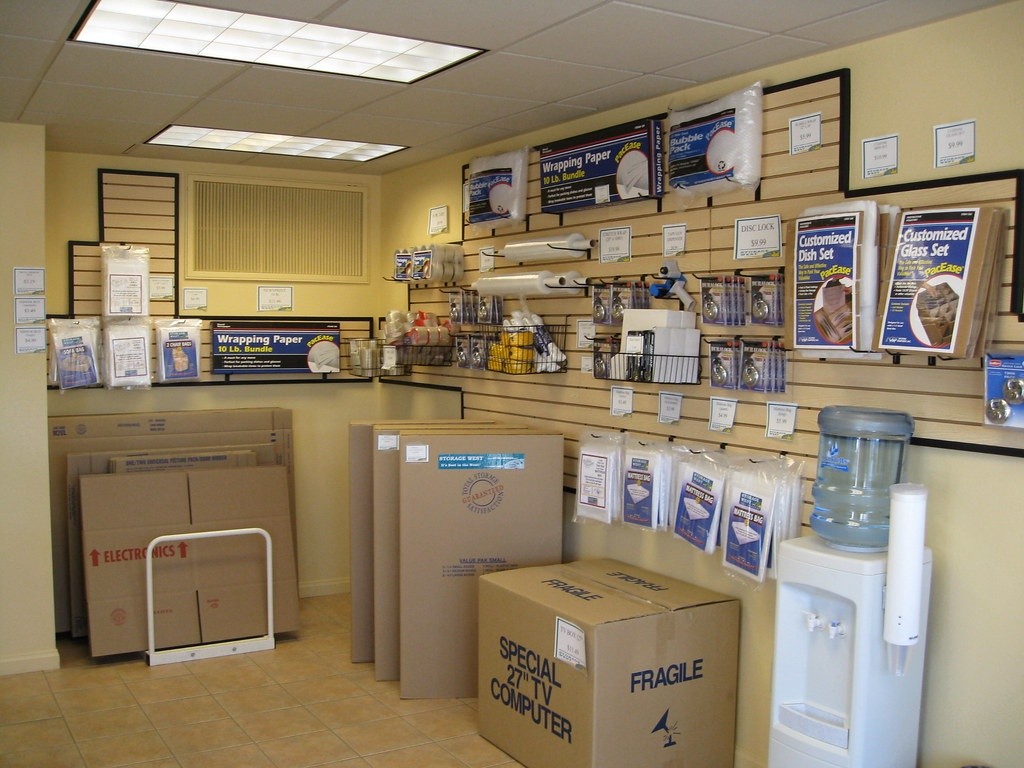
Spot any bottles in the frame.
[810,406,914,548]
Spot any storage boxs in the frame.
[620,308,700,383]
[540,120,664,212]
[477,558,741,768]
[347,418,563,699]
[209,322,341,375]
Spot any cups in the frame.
[883,484,928,677]
[350,339,378,375]
[361,349,380,377]
